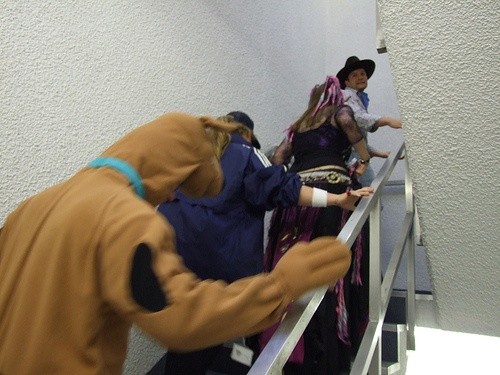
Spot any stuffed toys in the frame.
[0,113,351,375]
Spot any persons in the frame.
[157,111,375,374]
[336,56,401,309]
[259,75,370,375]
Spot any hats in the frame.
[228,111,261,150]
[336,56,376,90]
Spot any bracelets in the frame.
[360,157,370,164]
